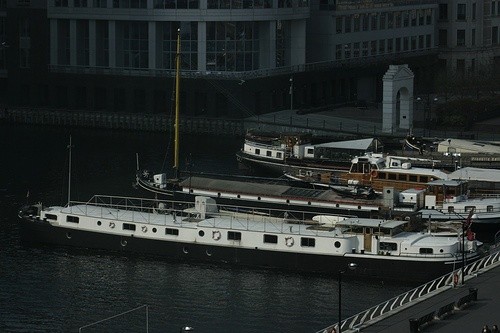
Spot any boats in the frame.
[235,76,500,198]
[14,136,484,286]
[131,27,500,244]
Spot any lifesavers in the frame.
[211,230,221,241]
[284,236,294,247]
[109,222,116,229]
[371,170,378,177]
[140,225,148,232]
